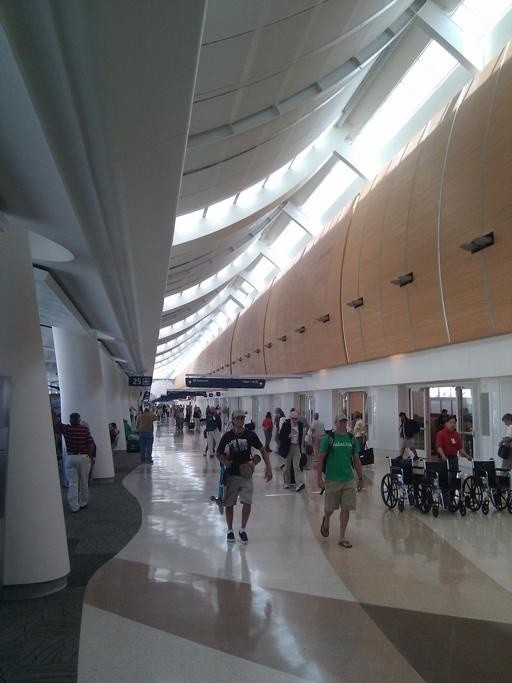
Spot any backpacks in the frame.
[409,419,418,433]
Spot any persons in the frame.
[314,412,365,548]
[497,412,511,492]
[433,414,473,512]
[123,400,324,494]
[435,407,448,458]
[56,411,99,512]
[396,410,421,462]
[458,414,473,457]
[215,408,274,543]
[351,409,368,452]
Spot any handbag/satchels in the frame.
[358,448,374,465]
[299,453,308,470]
[498,445,510,458]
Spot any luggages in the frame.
[284,463,303,484]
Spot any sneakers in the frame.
[284,484,291,489]
[296,484,305,491]
[142,460,153,464]
[71,503,87,513]
[239,530,249,543]
[227,532,235,542]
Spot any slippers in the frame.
[320,517,329,538]
[339,540,353,548]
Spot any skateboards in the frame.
[210,443,233,515]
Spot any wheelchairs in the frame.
[380,455,430,512]
[415,458,465,517]
[462,458,512,514]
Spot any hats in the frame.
[232,409,247,418]
[335,413,348,421]
[289,411,299,418]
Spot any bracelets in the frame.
[358,477,363,481]
